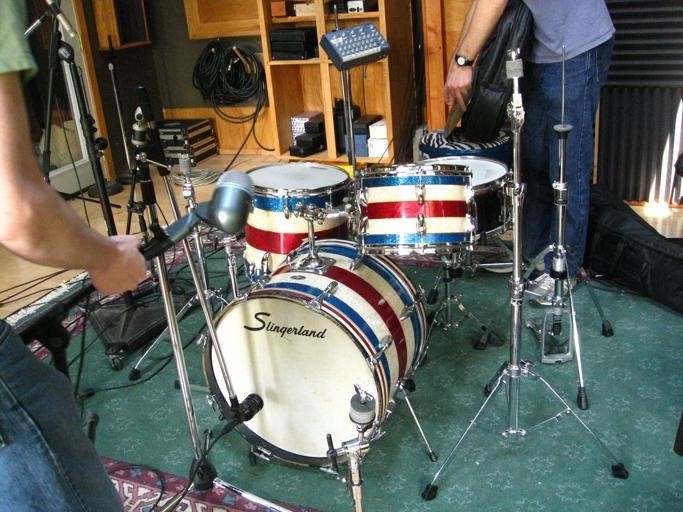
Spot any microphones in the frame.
[44,0,78,38]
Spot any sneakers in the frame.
[485,251,526,274]
[527,273,584,308]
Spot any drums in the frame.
[420,156,511,241]
[203,238,427,465]
[352,162,478,265]
[241,162,351,283]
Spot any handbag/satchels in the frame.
[457,0,529,142]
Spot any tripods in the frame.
[127,88,286,507]
[416,35,629,500]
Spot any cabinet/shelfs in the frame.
[163,0,410,165]
[92,0,153,53]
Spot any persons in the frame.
[440,0,615,305]
[0,0,149,512]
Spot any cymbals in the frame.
[443,86,471,138]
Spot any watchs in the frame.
[453,52,474,68]
